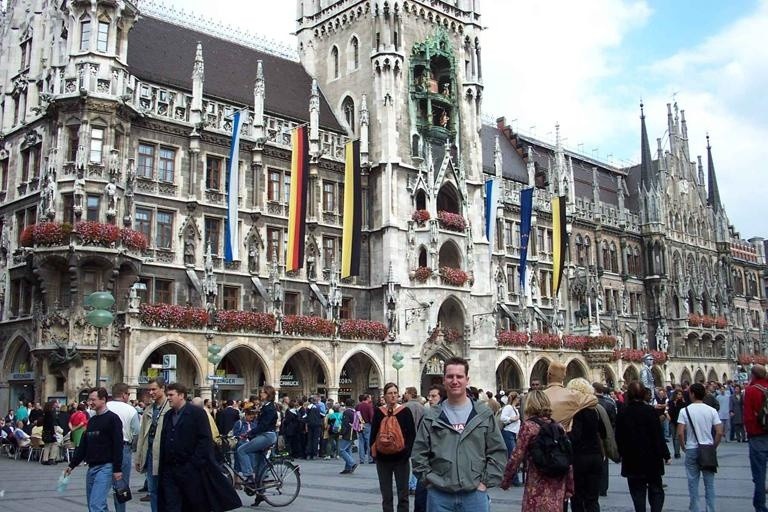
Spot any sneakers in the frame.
[665,454,673,465]
[335,455,340,460]
[674,453,681,458]
[340,463,359,474]
[323,455,331,460]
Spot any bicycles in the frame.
[214,433,303,507]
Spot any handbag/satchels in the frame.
[226,419,243,449]
[323,429,330,440]
[594,403,623,464]
[277,435,286,451]
[697,441,720,473]
[55,470,72,496]
[495,414,505,431]
[112,477,132,505]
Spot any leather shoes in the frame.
[138,487,148,492]
[140,495,150,501]
[239,477,255,485]
[251,496,264,507]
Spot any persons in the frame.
[741,362,768,511]
[639,353,656,401]
[410,356,508,512]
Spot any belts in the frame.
[123,440,133,446]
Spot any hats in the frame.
[500,390,505,396]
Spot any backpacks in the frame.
[347,408,366,432]
[753,384,768,431]
[372,403,406,456]
[529,415,573,478]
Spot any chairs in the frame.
[0,421,75,467]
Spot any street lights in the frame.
[87,286,116,401]
[392,351,404,401]
[206,343,223,413]
